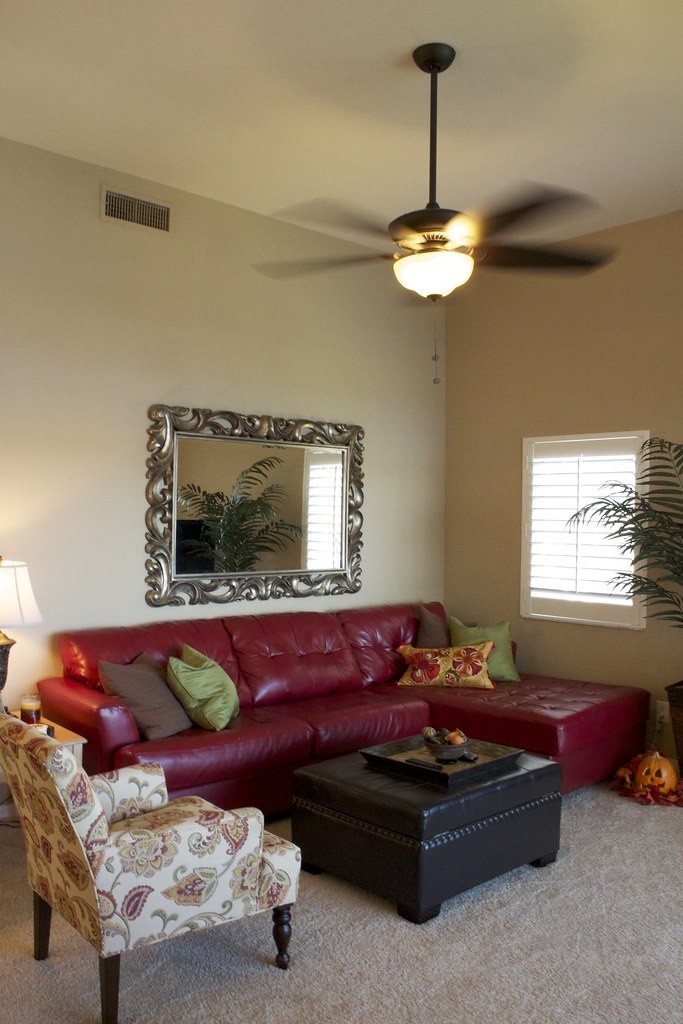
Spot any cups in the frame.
[21,693,41,724]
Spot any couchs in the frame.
[38,601,651,816]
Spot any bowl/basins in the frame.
[423,737,469,760]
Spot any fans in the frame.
[258,43,617,275]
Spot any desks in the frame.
[0,711,87,819]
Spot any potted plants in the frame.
[565,437,683,778]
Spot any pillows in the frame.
[397,604,521,689]
[98,643,239,741]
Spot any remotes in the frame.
[461,752,478,763]
[406,758,444,770]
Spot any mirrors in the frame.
[145,404,365,608]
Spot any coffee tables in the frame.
[292,734,562,924]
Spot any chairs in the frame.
[0,713,302,1024]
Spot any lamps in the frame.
[0,555,44,716]
[393,241,475,384]
